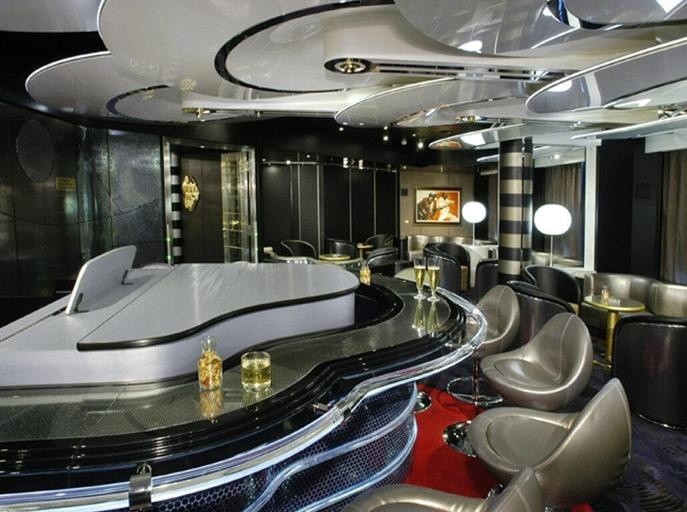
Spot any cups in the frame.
[240,351,272,393]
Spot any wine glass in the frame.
[411,299,442,332]
[426,258,441,303]
[413,256,427,300]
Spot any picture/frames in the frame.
[414,186,462,224]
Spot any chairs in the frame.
[464,377,634,512]
[263,227,498,292]
[400,270,686,462]
[333,467,544,511]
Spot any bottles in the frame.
[601,285,609,301]
[197,335,223,391]
[359,257,371,286]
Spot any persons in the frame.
[417,192,457,220]
